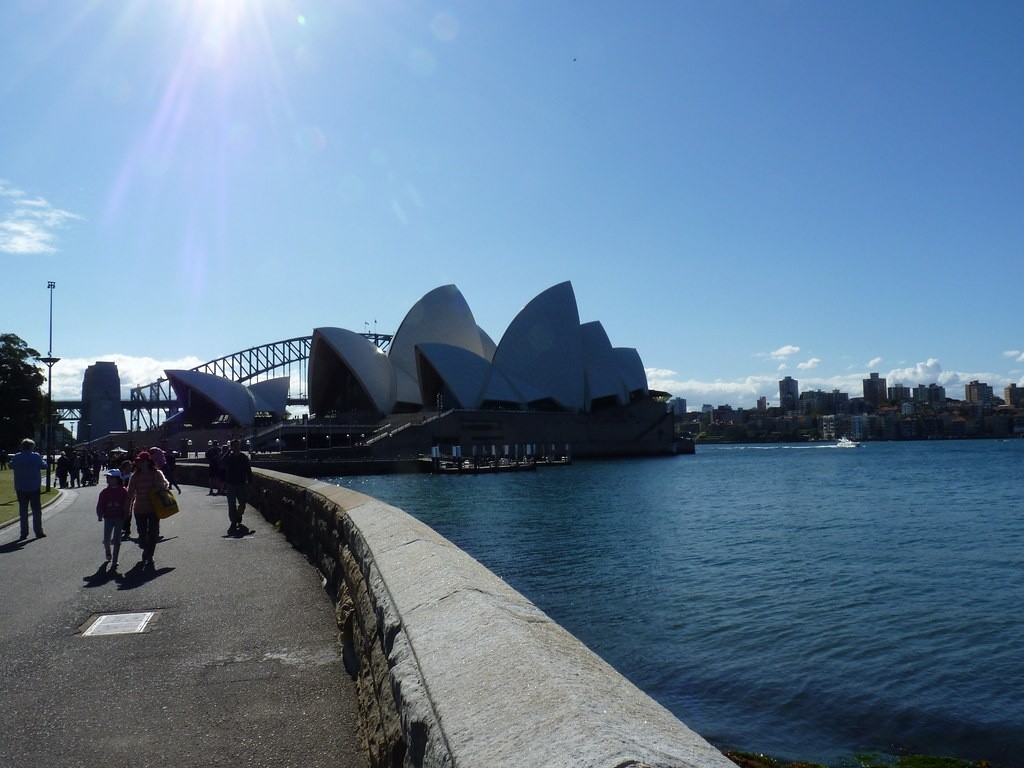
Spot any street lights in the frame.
[87,424,93,449]
[52,412,62,471]
[37,355,58,493]
[46,281,57,356]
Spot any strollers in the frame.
[53,467,69,489]
[88,467,98,486]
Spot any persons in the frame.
[56,446,181,541]
[8,439,48,539]
[96,469,130,566]
[127,452,168,564]
[0,450,14,471]
[205,440,252,523]
[195,449,198,458]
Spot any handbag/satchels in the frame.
[153,490,179,519]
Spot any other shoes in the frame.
[36,534,46,537]
[20,533,29,540]
[106,550,111,560]
[113,560,117,566]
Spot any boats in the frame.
[836,437,861,448]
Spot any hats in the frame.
[104,469,121,477]
[135,452,151,460]
